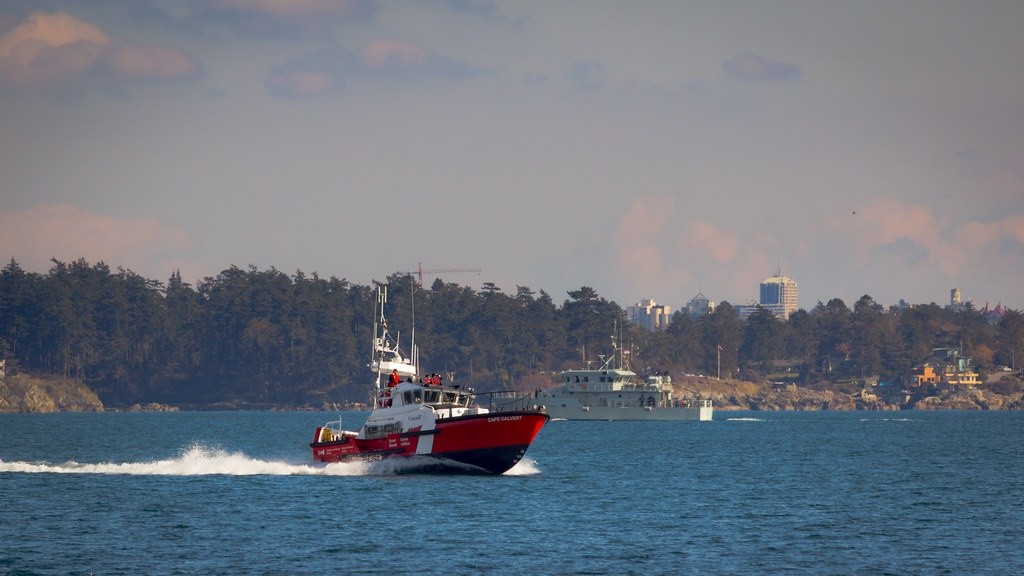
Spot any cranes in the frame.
[409,263,483,289]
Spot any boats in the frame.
[309,270,552,477]
[494,313,715,421]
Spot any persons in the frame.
[380,368,443,408]
